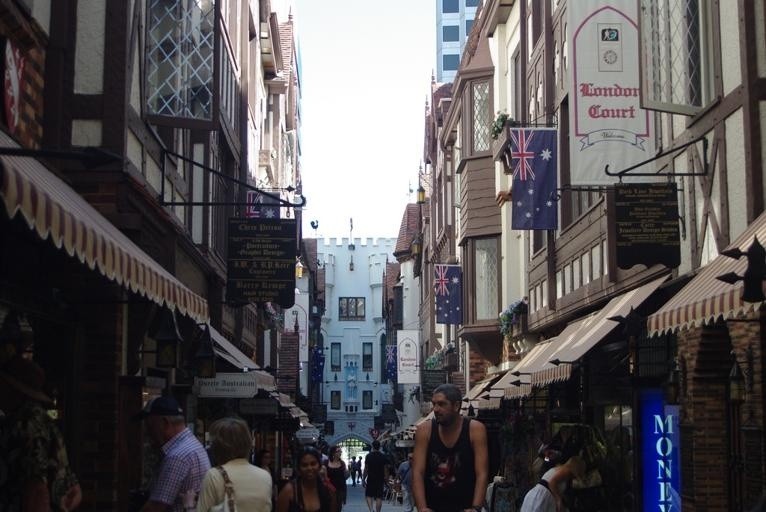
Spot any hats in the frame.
[130,397,183,422]
[0,357,52,404]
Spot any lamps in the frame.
[416,186,426,205]
[295,255,303,279]
[727,359,751,406]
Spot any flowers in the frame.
[489,109,513,140]
[497,296,530,345]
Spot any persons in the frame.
[517,417,634,512]
[393,452,413,512]
[181,415,274,511]
[362,439,389,512]
[252,441,366,512]
[410,383,492,511]
[0,358,82,512]
[128,395,213,511]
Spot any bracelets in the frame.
[470,504,482,511]
[186,504,195,510]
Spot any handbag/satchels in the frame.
[570,446,602,490]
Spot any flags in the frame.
[243,188,282,220]
[385,345,398,380]
[507,124,561,231]
[432,267,465,326]
